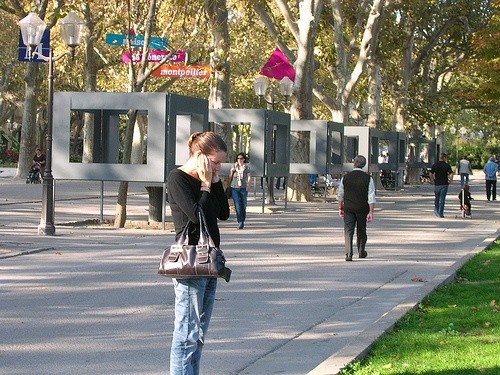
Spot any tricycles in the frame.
[454,187,473,220]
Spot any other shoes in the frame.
[359,251,367,258]
[346,253,353,261]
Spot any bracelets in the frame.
[339,208,343,210]
[201,185,210,189]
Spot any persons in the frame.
[33,147,46,178]
[225,153,253,229]
[429,153,454,218]
[336,155,376,261]
[163,130,230,375]
[483,153,500,202]
[457,156,475,215]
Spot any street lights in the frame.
[253,76,294,204]
[347,99,373,158]
[18,10,86,237]
[449,125,468,175]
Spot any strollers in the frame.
[25,161,44,185]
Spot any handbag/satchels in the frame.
[225,187,232,199]
[157,205,232,283]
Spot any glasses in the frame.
[237,158,244,159]
